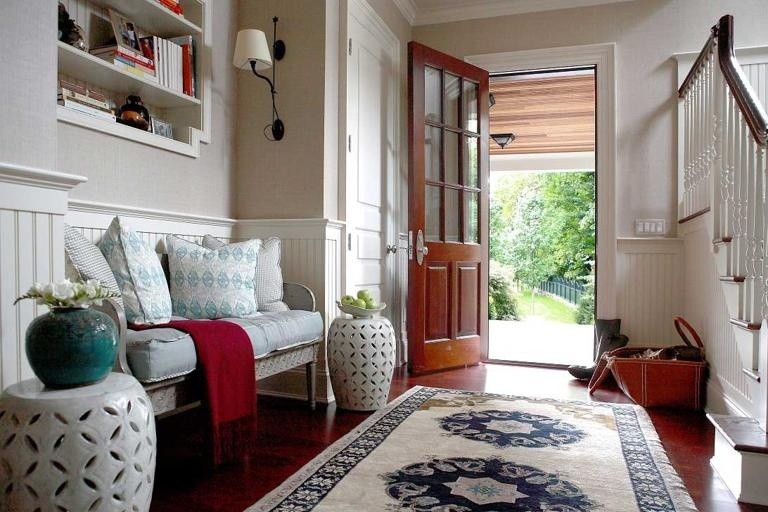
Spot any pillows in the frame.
[98,213,172,324]
[202,234,290,312]
[62,222,126,313]
[166,232,265,319]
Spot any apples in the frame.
[341,289,377,309]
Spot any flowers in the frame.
[13,275,123,310]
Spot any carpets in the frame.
[244,383,699,510]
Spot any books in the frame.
[58,0,200,138]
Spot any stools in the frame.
[327,316,397,413]
[0,371,158,512]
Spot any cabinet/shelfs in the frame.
[58,0,212,158]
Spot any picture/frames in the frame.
[106,8,145,57]
[149,116,174,141]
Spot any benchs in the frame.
[62,220,324,419]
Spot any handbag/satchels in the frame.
[606,345,707,410]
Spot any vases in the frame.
[25,307,120,390]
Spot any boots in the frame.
[567,319,629,384]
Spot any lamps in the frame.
[232,17,289,143]
[488,93,496,110]
[490,133,515,150]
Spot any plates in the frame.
[336,303,387,318]
